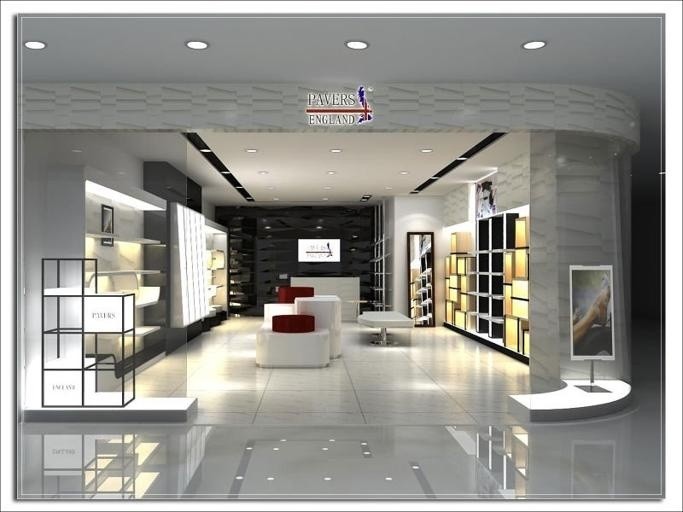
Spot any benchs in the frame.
[356,310,412,347]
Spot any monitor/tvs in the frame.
[298,239,341,263]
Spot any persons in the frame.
[573,273,611,343]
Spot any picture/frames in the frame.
[101,204,113,245]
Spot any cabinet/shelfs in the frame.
[206,250,254,316]
[85,230,165,335]
[411,246,431,324]
[440,204,529,364]
[369,235,391,310]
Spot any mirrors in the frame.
[406,232,435,327]
